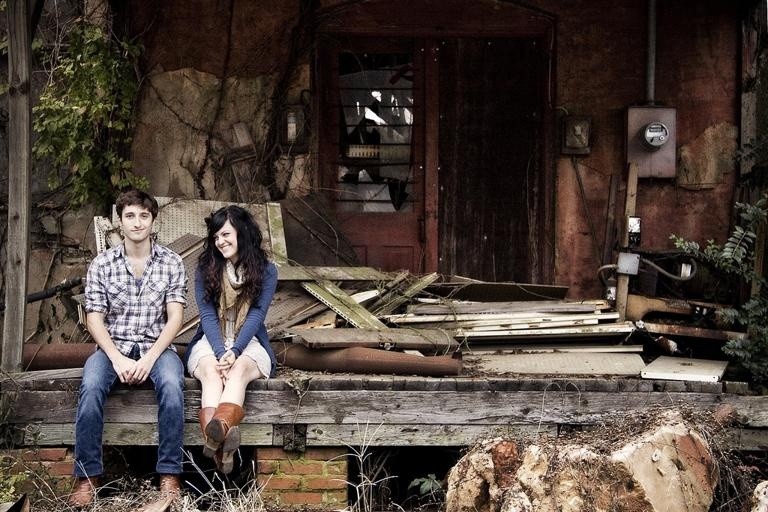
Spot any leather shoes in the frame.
[160,475,180,512]
[70,475,99,505]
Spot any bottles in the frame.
[288,112,298,147]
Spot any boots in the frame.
[198,403,245,474]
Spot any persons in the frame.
[184,205,279,475]
[70,189,189,510]
